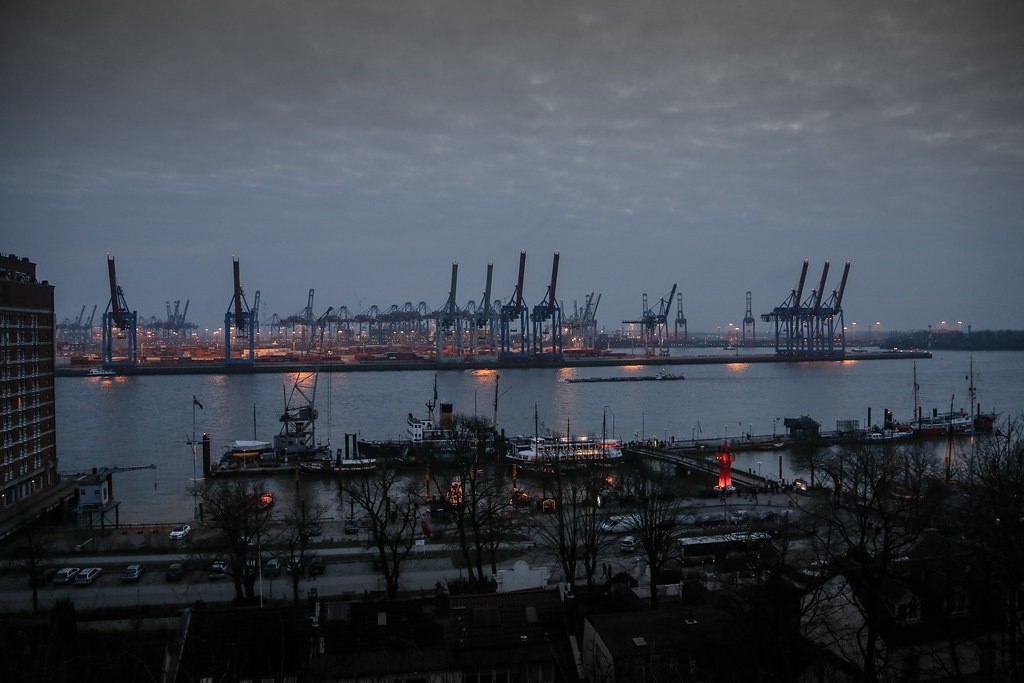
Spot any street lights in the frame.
[664,429,668,441]
[725,425,727,440]
[758,461,762,476]
[692,426,695,439]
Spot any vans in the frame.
[602,516,642,533]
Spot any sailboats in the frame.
[904,361,972,429]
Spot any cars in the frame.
[265,559,282,575]
[242,560,255,575]
[121,565,145,581]
[75,568,102,585]
[166,563,183,581]
[54,568,80,584]
[287,556,302,573]
[309,524,320,536]
[209,561,227,577]
[345,519,355,532]
[169,525,191,539]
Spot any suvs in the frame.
[621,537,638,550]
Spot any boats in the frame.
[861,424,913,442]
[299,457,383,473]
[356,374,497,456]
[505,402,623,470]
[233,402,272,450]
[86,369,117,376]
[254,491,274,511]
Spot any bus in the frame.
[676,531,775,566]
